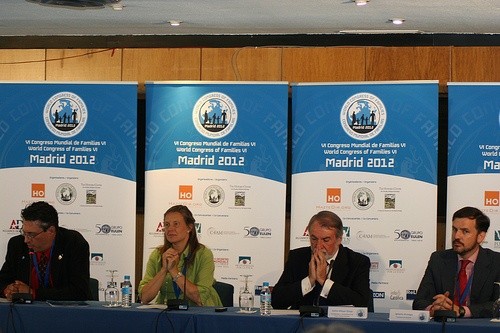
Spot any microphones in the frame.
[434,252,456,322]
[13,248,35,303]
[167,257,188,311]
[300,260,334,316]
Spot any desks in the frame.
[0,298,500,333]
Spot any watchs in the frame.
[173,271,183,282]
[457,306,465,319]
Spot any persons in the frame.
[411,206,500,320]
[0,201,93,303]
[270,210,375,317]
[135,204,224,309]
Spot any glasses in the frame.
[19,227,45,239]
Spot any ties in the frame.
[36,254,52,291]
[453,260,470,307]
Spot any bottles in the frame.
[259,282,272,317]
[121,276,133,308]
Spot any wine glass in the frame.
[104,269,120,308]
[491,281,500,322]
[238,274,255,313]
